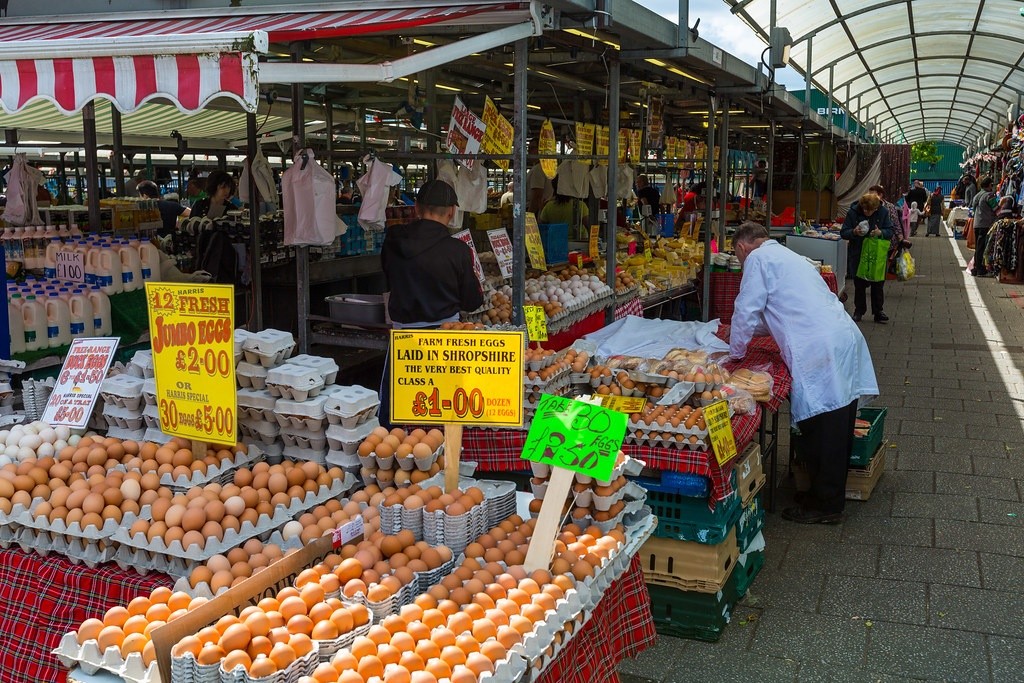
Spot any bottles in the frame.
[0,224,162,353]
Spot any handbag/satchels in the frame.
[885,241,915,282]
[924,198,931,216]
[857,233,891,281]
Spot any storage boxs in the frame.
[732,438,767,506]
[701,187,717,197]
[734,491,768,555]
[791,405,889,465]
[69,206,113,231]
[848,249,861,274]
[784,228,850,297]
[540,217,570,264]
[650,567,737,649]
[640,469,743,546]
[325,292,385,324]
[37,206,70,226]
[100,199,136,231]
[475,213,498,231]
[735,531,771,598]
[638,525,739,594]
[138,218,164,231]
[790,441,891,502]
[655,213,675,237]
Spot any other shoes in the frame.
[781,503,843,525]
[874,310,890,321]
[852,310,863,321]
[977,268,994,278]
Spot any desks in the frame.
[0,480,676,683]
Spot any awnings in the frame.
[0,0,556,117]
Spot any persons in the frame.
[840,193,895,323]
[336,178,417,206]
[38,169,245,239]
[729,222,864,524]
[962,176,976,207]
[906,180,945,237]
[376,179,484,434]
[970,176,1008,277]
[486,137,772,242]
[870,184,904,271]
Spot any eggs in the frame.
[439,250,636,331]
[358,427,446,486]
[77,484,627,683]
[0,421,343,567]
[523,346,722,447]
[528,449,628,522]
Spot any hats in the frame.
[415,179,460,207]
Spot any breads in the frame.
[661,350,773,403]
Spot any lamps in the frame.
[793,122,805,128]
[739,124,783,129]
[770,25,793,69]
[645,57,715,88]
[685,107,747,115]
[560,24,621,51]
